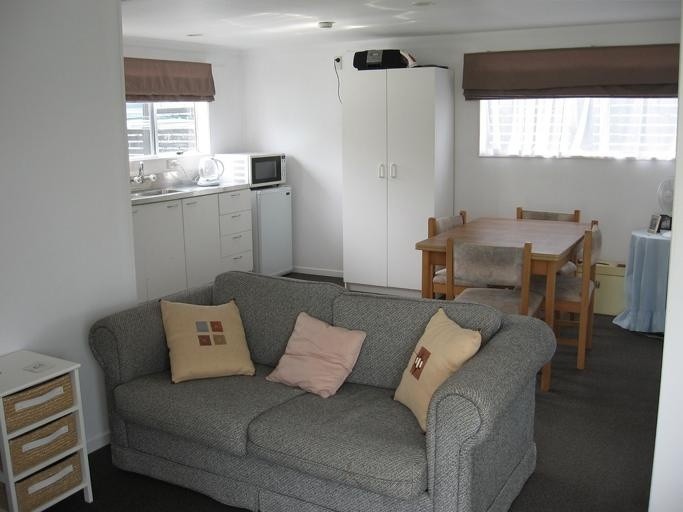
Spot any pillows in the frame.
[393,308,482,431]
[158,299,258,385]
[266,311,366,399]
[212,271,344,369]
[333,291,501,391]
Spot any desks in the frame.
[612,228,672,334]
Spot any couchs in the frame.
[88,283,557,512]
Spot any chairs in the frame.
[428,209,492,298]
[446,238,544,319]
[514,220,602,370]
[516,207,580,321]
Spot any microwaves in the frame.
[214,152,287,189]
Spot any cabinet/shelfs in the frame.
[0,364,94,512]
[341,68,455,297]
[132,185,254,307]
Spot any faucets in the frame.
[137,159,145,175]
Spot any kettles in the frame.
[196,157,225,186]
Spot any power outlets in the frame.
[334,56,342,65]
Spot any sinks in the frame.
[131,188,179,198]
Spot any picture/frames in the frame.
[647,215,661,234]
